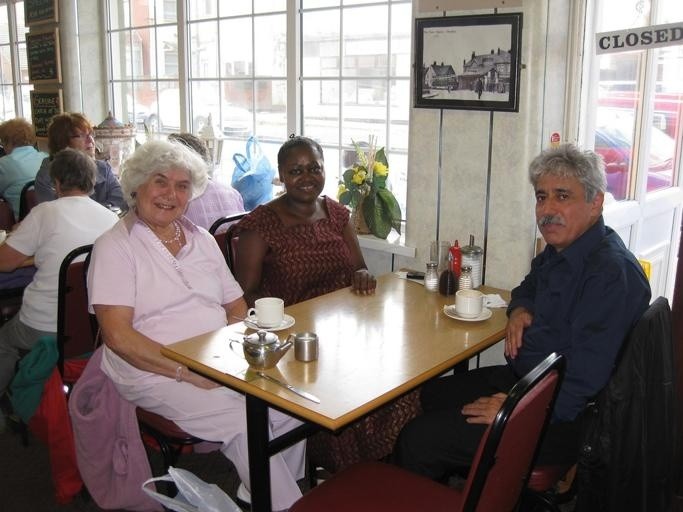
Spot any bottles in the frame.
[423,236,482,295]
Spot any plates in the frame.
[441,304,492,324]
[243,315,294,331]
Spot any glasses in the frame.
[66,131,98,141]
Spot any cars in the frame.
[590,78,681,201]
[147,87,254,140]
[112,91,146,126]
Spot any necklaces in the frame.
[158,220,180,244]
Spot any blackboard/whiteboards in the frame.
[24,0,59,27]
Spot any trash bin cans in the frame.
[342,141,372,169]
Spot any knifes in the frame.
[257,371,321,403]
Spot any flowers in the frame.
[334,131,402,242]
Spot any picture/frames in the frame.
[21,0,60,29]
[409,9,524,114]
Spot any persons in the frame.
[476,78,483,99]
[393,142,645,486]
[167,133,245,238]
[34,112,129,214]
[233,135,424,467]
[0,118,46,220]
[97,131,319,511]
[0,147,123,398]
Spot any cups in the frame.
[0,229,6,246]
[453,289,487,319]
[295,331,319,362]
[247,297,287,328]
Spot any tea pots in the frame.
[228,330,294,370]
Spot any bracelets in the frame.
[173,366,182,382]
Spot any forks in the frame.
[230,314,274,330]
